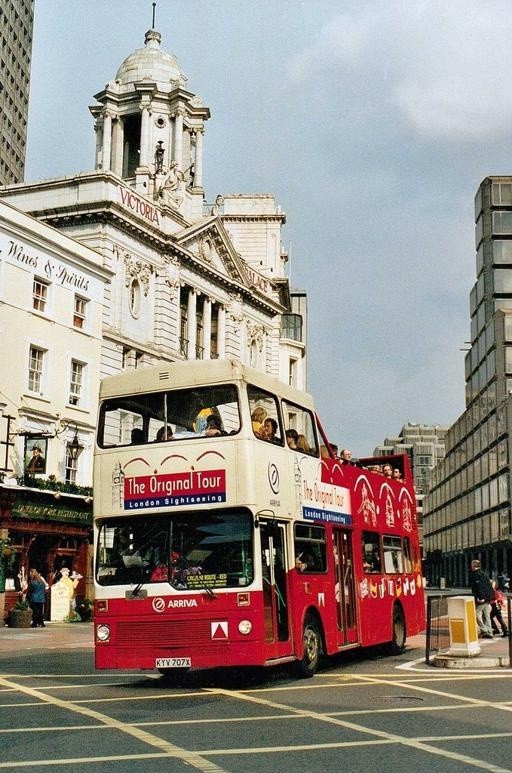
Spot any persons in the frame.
[490,579,509,637]
[27,447,45,472]
[26,569,46,627]
[471,560,496,638]
[129,408,405,484]
[17,576,49,596]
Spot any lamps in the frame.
[53,484,62,500]
[55,419,85,459]
[83,490,91,503]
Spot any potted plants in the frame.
[5,601,33,627]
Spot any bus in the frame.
[87,357,431,676]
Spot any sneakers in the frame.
[478,632,492,639]
[492,628,499,635]
[500,630,509,639]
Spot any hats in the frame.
[59,568,70,573]
